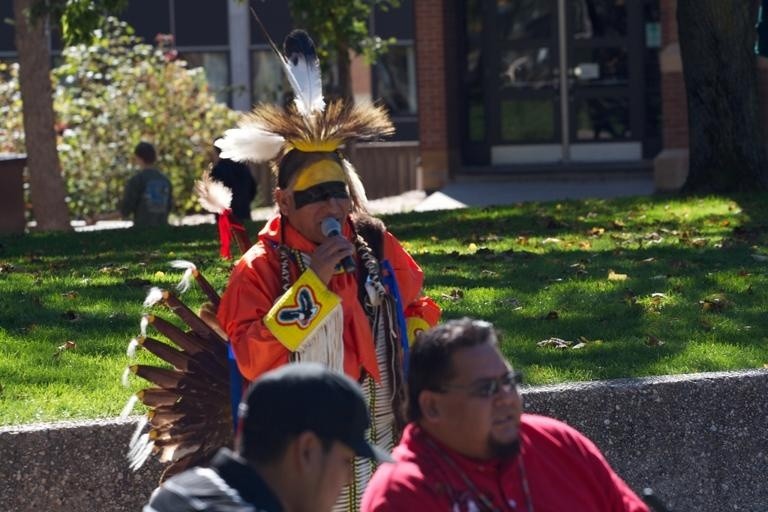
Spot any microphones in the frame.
[320,216,356,272]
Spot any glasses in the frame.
[442,372,522,397]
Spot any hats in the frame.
[247,362,395,462]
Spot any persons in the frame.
[214,147,446,512]
[112,139,174,232]
[155,35,191,68]
[141,358,401,512]
[358,317,650,512]
[209,135,260,223]
